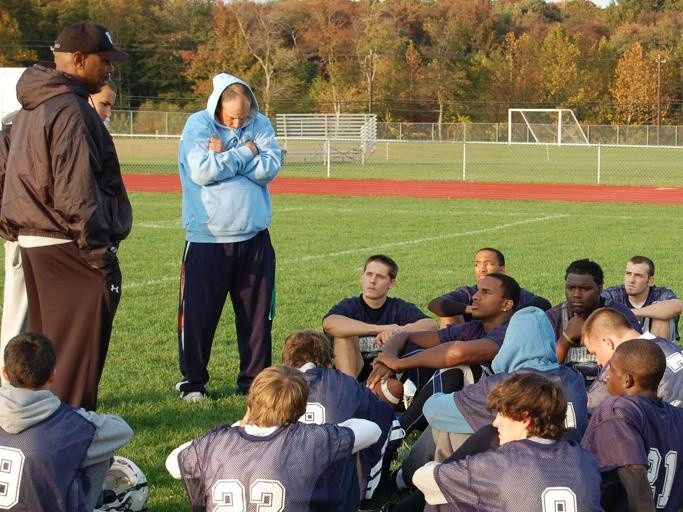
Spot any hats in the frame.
[49,21,131,64]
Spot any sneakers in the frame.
[183,391,203,403]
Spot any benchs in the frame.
[286,151,361,163]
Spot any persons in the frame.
[282,247,683,511]
[173,70,283,405]
[0,78,118,369]
[0,20,132,411]
[163,360,382,511]
[0,329,134,511]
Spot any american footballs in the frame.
[367,379,404,407]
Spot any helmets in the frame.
[91,456,149,512]
